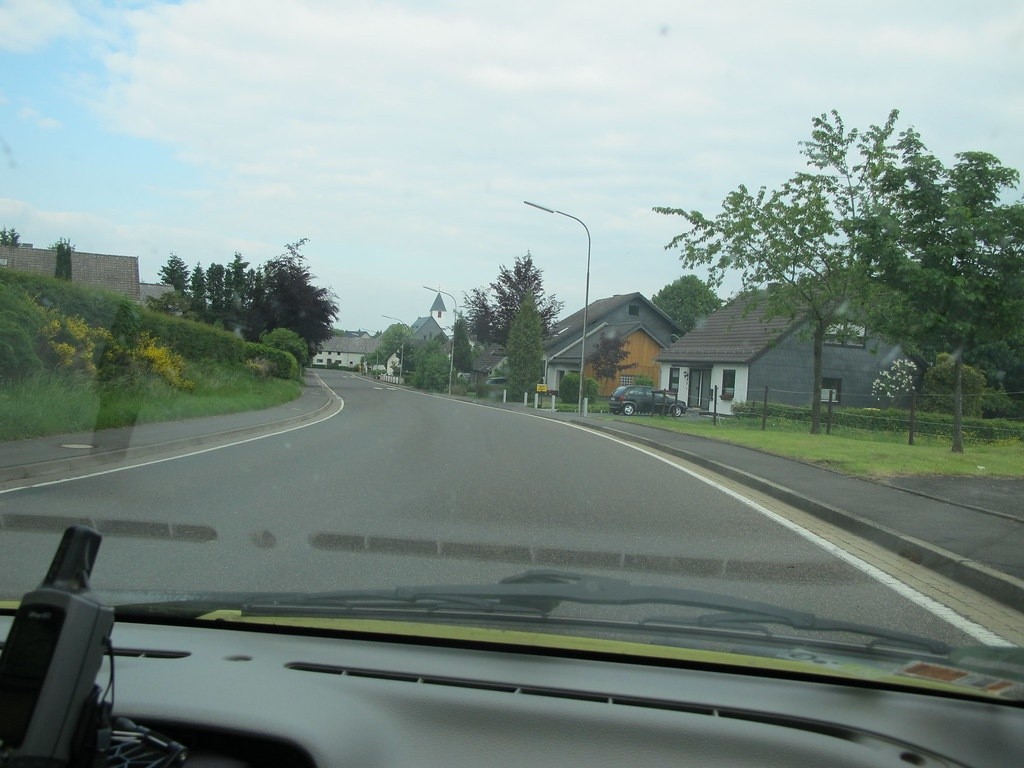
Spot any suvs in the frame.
[607,385,688,417]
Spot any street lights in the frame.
[381,313,404,386]
[422,285,458,396]
[522,199,592,416]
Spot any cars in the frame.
[485,378,506,389]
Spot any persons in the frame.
[361,359,368,377]
